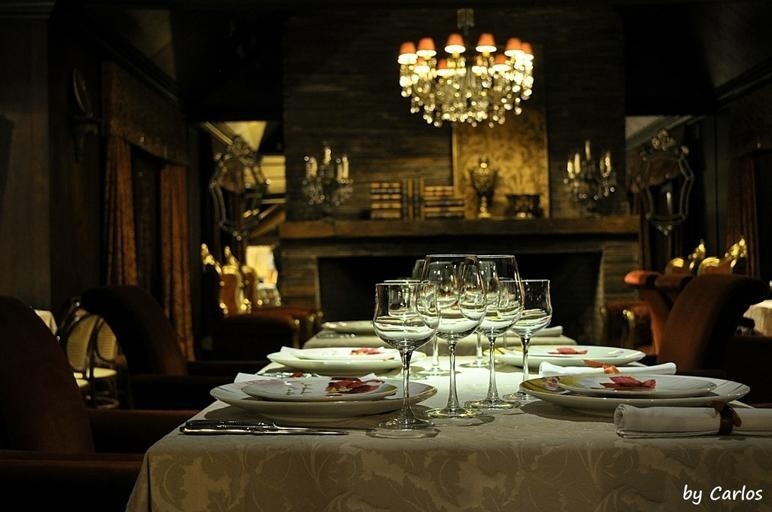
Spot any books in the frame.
[369,178,465,219]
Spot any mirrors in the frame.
[193,71,283,245]
[626,113,718,272]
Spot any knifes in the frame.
[187,419,372,431]
[178,425,349,436]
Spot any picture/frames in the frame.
[450,49,551,218]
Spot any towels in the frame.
[614,405,771,438]
[537,360,676,376]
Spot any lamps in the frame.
[567,139,615,219]
[396,9,535,130]
[304,148,354,219]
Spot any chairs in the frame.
[623,271,668,324]
[201,244,311,377]
[83,315,119,411]
[54,309,99,408]
[226,245,322,341]
[0,294,200,512]
[655,274,693,305]
[661,275,771,370]
[82,286,264,409]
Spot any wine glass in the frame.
[366,283,439,438]
[420,255,486,418]
[465,254,522,409]
[461,261,499,368]
[413,260,458,376]
[503,280,552,402]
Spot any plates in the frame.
[557,379,716,398]
[266,347,427,377]
[499,345,647,368]
[320,320,376,335]
[209,383,437,422]
[519,375,750,417]
[242,383,397,402]
